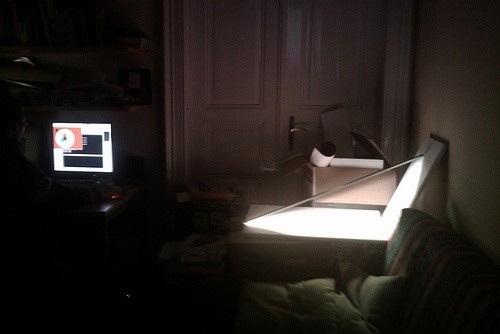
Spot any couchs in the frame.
[226,206,500,334]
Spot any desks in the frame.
[42,189,147,277]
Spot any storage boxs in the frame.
[299,162,398,209]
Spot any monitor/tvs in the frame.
[48,118,117,178]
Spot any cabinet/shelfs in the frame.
[0,45,155,112]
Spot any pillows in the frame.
[234,277,377,334]
[354,276,409,334]
